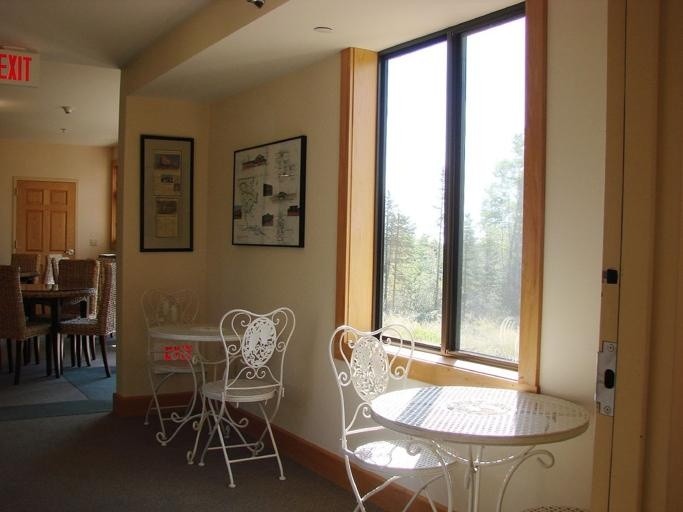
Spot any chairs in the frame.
[1,253,116,385]
[198,308,297,487]
[142,287,215,435]
[328,325,457,512]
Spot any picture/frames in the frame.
[232,134,307,248]
[139,133,193,253]
